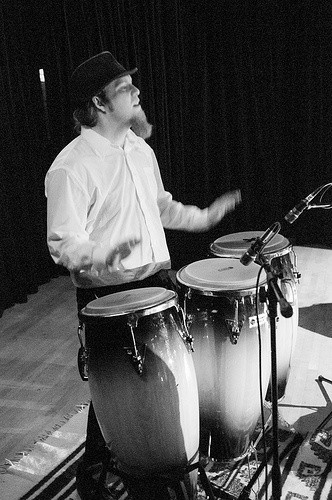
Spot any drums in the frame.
[174,257,272,460]
[208,231,298,407]
[76,287,202,499]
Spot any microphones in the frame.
[285,184,328,224]
[240,223,279,266]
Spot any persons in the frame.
[43,49,242,499]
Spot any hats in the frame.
[60,50,139,123]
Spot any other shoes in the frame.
[77,477,116,500]
